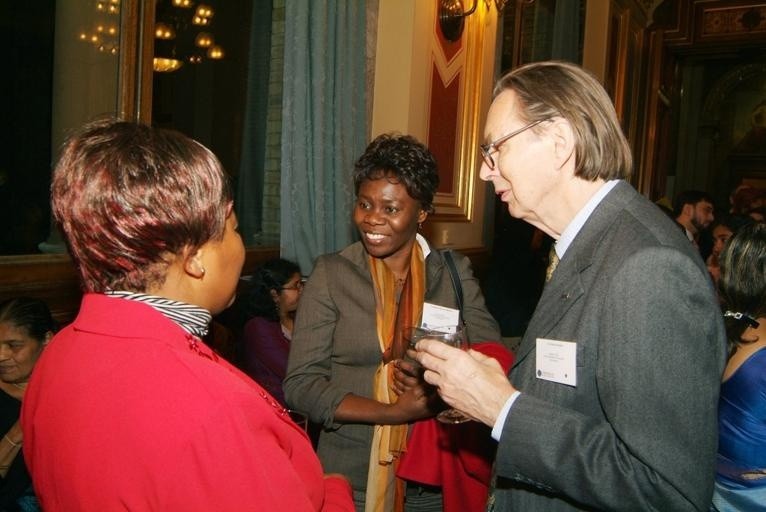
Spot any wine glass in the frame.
[403,325,473,423]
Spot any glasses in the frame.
[480,119,537,171]
[281,282,304,291]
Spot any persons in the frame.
[402,58,729,512]
[745,207,766,225]
[704,213,755,304]
[237,257,305,424]
[20,116,358,512]
[280,128,503,512]
[671,190,715,234]
[709,224,766,512]
[0,295,68,512]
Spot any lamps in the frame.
[439,0,507,42]
